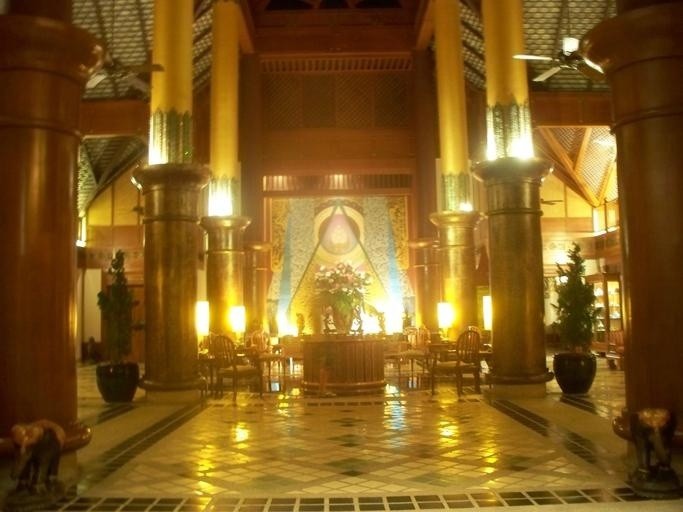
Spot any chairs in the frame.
[380,322,483,399]
[206,328,304,404]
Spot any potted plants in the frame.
[93,247,140,404]
[547,240,604,394]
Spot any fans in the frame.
[85,0,166,99]
[510,1,605,86]
